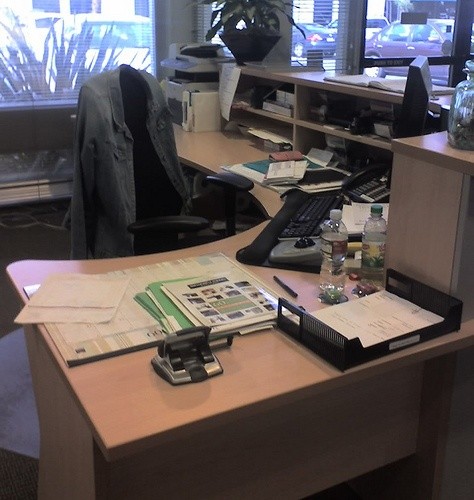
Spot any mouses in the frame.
[285,190,308,206]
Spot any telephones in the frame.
[340,162,390,203]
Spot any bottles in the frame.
[359,205,387,295]
[447,58,474,151]
[320,209,348,303]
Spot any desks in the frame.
[164,126,289,220]
[6,128,474,500]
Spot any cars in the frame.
[291,22,335,58]
[46,13,152,90]
[364,20,474,86]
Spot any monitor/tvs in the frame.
[385,54,434,190]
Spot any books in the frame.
[134,267,293,337]
[323,74,456,94]
[263,160,309,186]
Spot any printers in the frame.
[159,41,235,131]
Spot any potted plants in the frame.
[179,0,306,62]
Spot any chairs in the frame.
[78,70,253,259]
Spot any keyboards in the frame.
[276,194,343,240]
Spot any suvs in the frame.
[326,14,390,39]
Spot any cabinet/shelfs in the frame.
[224,65,449,185]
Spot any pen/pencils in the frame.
[273,276,298,298]
